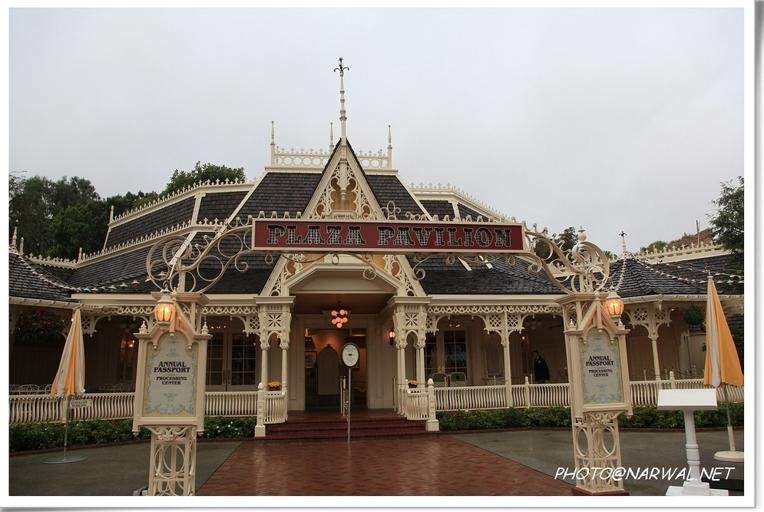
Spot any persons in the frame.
[530,348,550,385]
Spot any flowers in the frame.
[268,382,281,388]
[408,380,417,386]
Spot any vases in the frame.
[270,386,280,391]
[409,383,417,388]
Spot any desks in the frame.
[482,377,504,385]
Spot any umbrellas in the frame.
[47,303,86,454]
[702,269,744,449]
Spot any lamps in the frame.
[331,301,351,329]
[154,286,176,332]
[448,320,464,328]
[389,327,395,345]
[520,331,530,344]
[602,285,624,322]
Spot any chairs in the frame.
[432,373,448,387]
[450,372,467,387]
[9,383,52,413]
